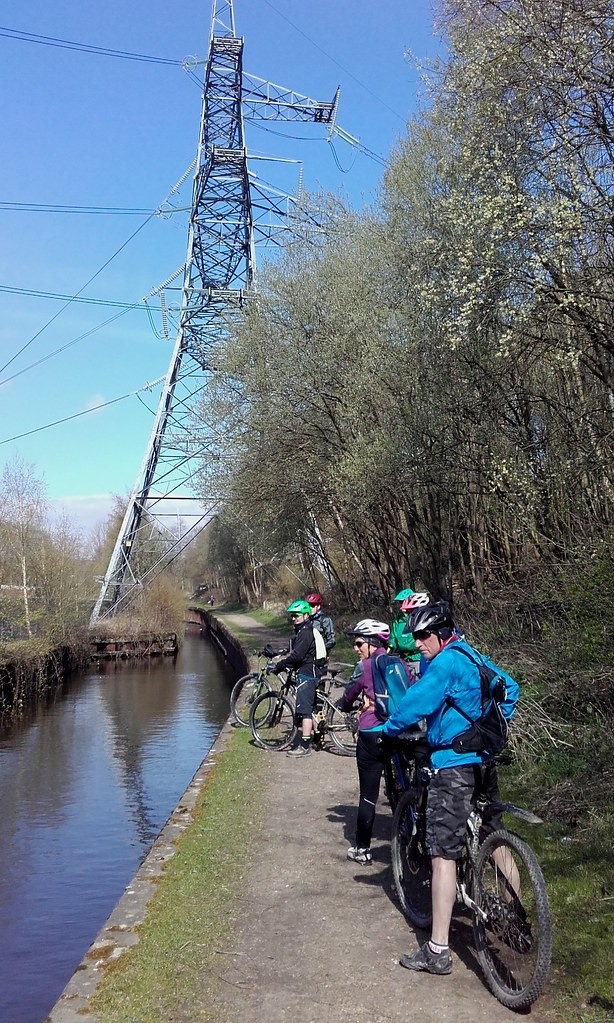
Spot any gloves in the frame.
[339,699,354,712]
[276,660,286,672]
[376,731,392,750]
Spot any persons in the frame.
[388,588,469,688]
[337,618,422,866]
[207,595,215,606]
[381,607,534,974]
[266,599,326,758]
[279,593,336,745]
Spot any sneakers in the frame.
[286,745,312,758]
[347,846,372,866]
[510,924,531,952]
[311,740,322,749]
[399,941,452,974]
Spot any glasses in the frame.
[291,614,301,617]
[310,603,318,608]
[352,640,366,648]
[412,629,432,641]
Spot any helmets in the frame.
[393,589,414,601]
[286,600,311,615]
[401,601,451,634]
[304,593,322,604]
[347,619,390,642]
[400,592,429,612]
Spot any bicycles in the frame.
[337,702,428,881]
[231,647,362,731]
[377,731,553,1010]
[247,661,368,756]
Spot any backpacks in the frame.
[370,654,411,722]
[444,646,509,752]
